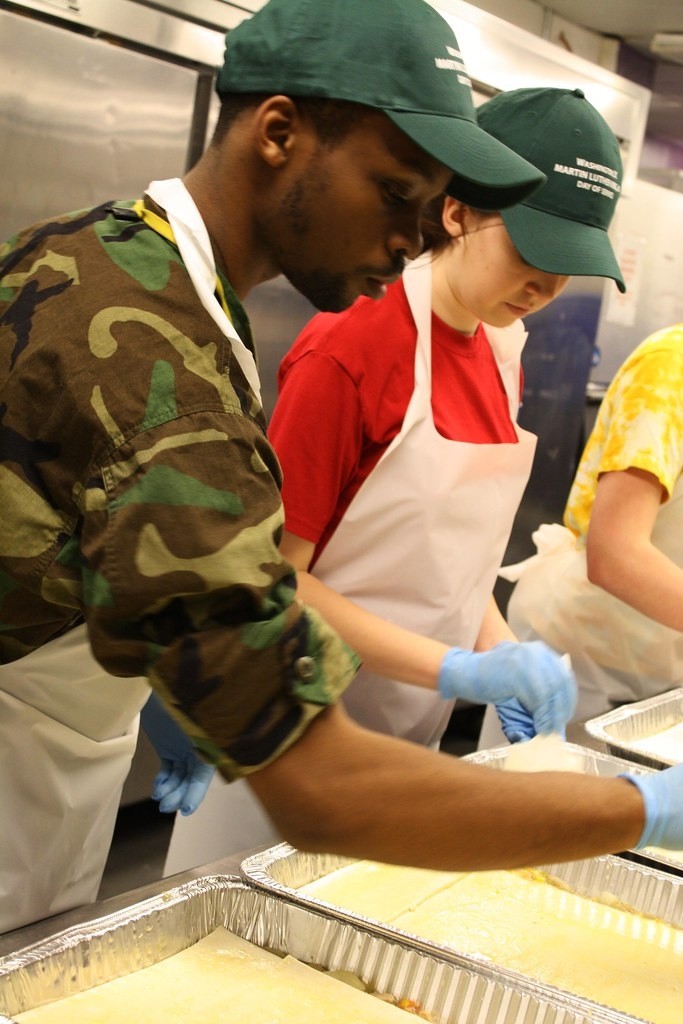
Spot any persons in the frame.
[478,322,683,755]
[0,1,682,937]
[157,89,628,883]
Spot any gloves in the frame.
[137,689,217,816]
[619,763,683,852]
[439,640,578,741]
[495,696,537,744]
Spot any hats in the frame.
[476,87,626,295]
[215,0,547,212]
